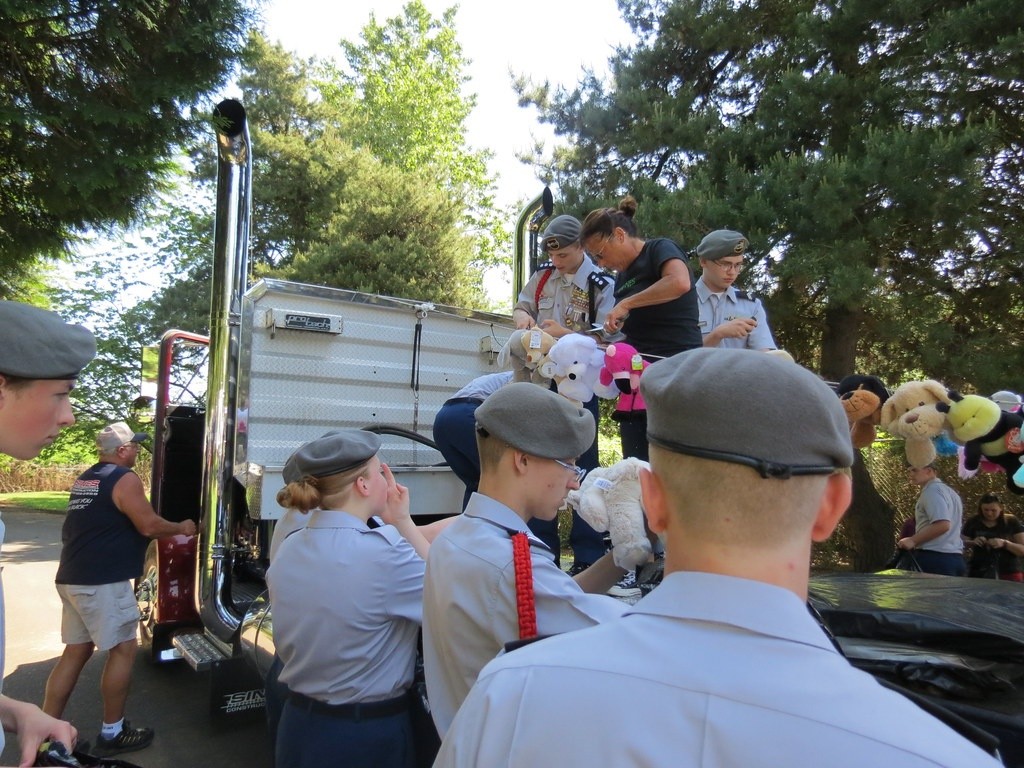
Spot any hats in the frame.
[0,300,98,378]
[697,230,749,260]
[474,382,595,459]
[904,460,943,470]
[540,214,583,251]
[96,422,147,451]
[282,428,382,483]
[640,347,854,476]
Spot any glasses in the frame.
[712,260,746,273]
[593,231,627,261]
[554,459,587,482]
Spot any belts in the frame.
[290,692,411,721]
[443,397,483,406]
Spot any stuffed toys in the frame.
[497,325,650,410]
[831,374,1024,495]
[567,457,652,571]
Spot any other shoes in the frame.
[565,562,591,577]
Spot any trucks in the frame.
[134,96,1024,768]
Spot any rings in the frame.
[604,322,610,325]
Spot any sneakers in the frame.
[74,738,90,753]
[606,570,642,596]
[92,719,154,758]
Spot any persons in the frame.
[896,461,1024,584]
[0,299,97,768]
[42,423,195,755]
[431,346,1001,768]
[266,429,461,768]
[434,195,781,599]
[424,381,657,746]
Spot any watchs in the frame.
[1003,540,1009,548]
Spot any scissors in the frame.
[585,313,630,335]
